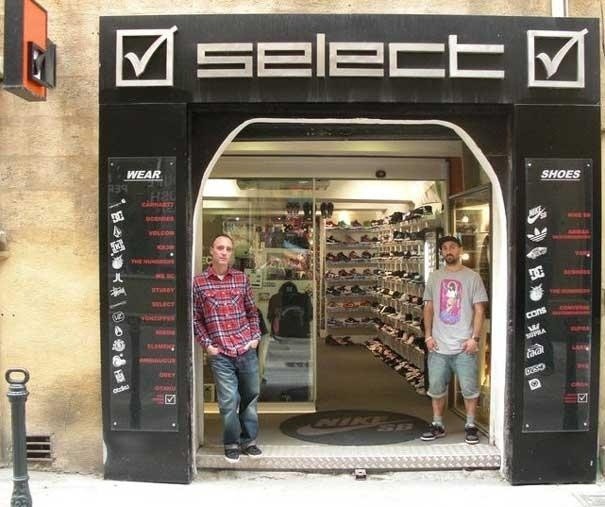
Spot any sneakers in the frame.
[465,423,481,444]
[223,444,264,462]
[282,201,431,396]
[419,423,447,440]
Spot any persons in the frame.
[193,234,261,463]
[421,236,488,444]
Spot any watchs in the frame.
[472,336,479,342]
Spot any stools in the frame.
[256,333,315,388]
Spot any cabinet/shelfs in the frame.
[324,207,492,408]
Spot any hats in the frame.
[438,235,462,251]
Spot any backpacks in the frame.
[267,281,313,338]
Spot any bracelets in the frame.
[424,336,432,343]
